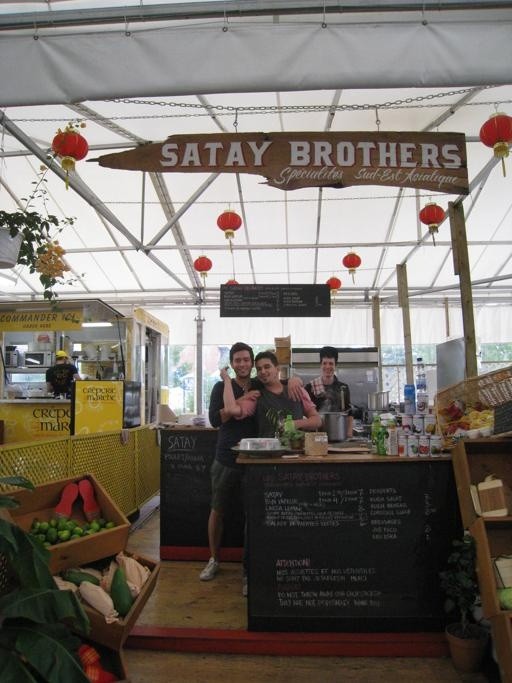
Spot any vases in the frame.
[0,228,24,269]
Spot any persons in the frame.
[301,346,354,438]
[46,350,82,396]
[219,351,323,594]
[198,341,304,580]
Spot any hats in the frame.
[56,351,66,358]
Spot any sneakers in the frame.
[200,556,219,580]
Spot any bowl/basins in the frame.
[108,357,114,360]
[6,346,17,350]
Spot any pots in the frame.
[317,411,350,441]
[367,389,390,411]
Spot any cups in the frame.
[7,390,15,400]
[240,437,281,450]
[60,393,66,399]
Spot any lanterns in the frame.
[342,251,361,283]
[479,103,512,177]
[327,275,341,302]
[53,124,89,190]
[194,253,213,284]
[216,207,242,253]
[226,280,240,283]
[418,199,445,247]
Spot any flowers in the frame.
[0,195,76,314]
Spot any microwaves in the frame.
[24,352,53,368]
[5,351,18,367]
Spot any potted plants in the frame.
[439,536,490,673]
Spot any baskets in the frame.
[436,367,512,447]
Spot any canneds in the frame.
[416,391,429,415]
[397,412,452,458]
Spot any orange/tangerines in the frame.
[28,516,115,548]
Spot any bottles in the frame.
[372,417,398,456]
[414,357,428,393]
[274,413,303,448]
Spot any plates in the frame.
[287,450,304,454]
[231,448,288,457]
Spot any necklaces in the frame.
[238,376,251,391]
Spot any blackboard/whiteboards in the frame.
[220,284,330,317]
